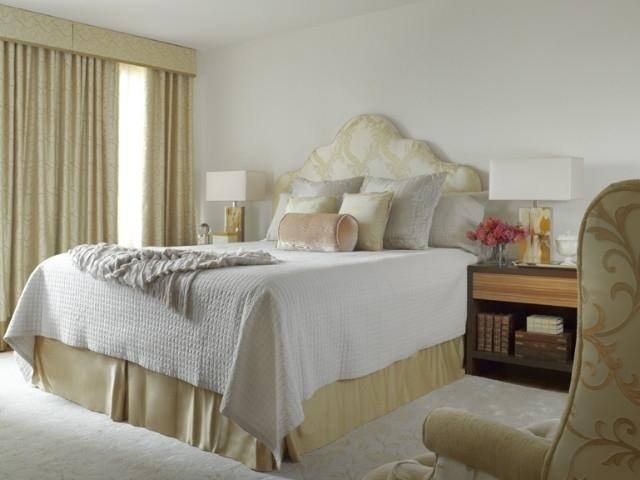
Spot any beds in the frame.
[3,114,482,473]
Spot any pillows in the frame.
[266,171,489,252]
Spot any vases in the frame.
[484,242,509,265]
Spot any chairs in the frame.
[364,180,640,480]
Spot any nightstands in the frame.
[466,263,577,376]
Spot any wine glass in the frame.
[555,230,578,266]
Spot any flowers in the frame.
[468,219,528,246]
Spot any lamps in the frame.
[488,158,583,264]
[207,171,273,241]
